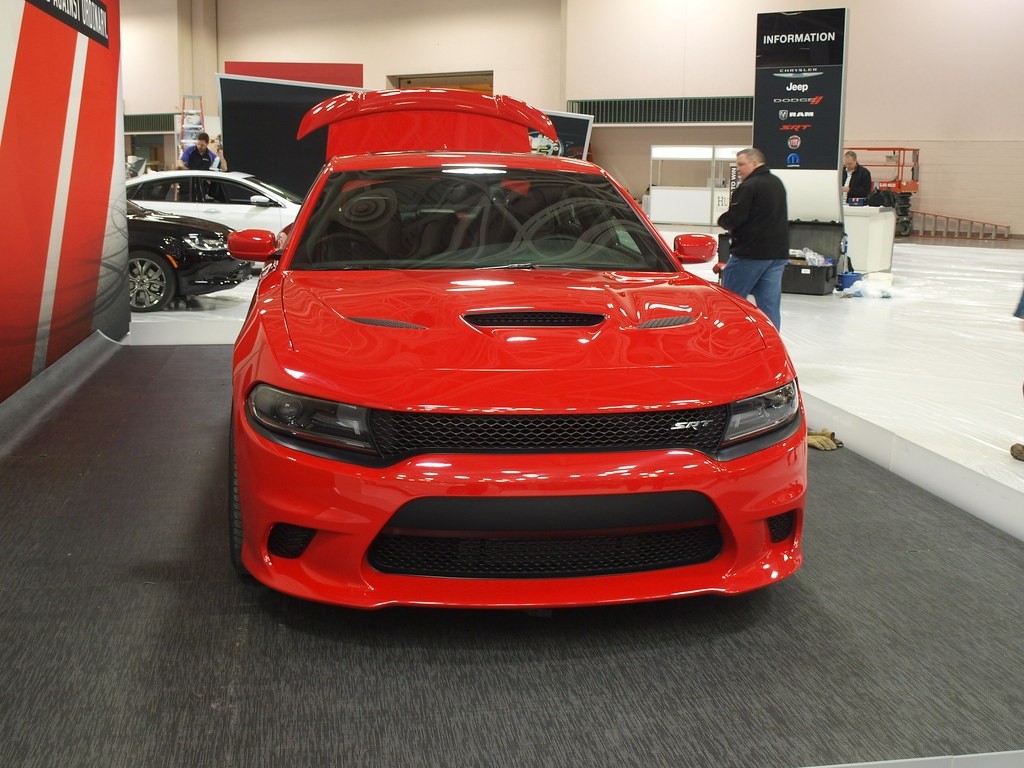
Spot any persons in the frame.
[718,149,788,333]
[1011,290,1024,461]
[842,151,872,203]
[177,132,228,171]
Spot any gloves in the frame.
[806,428,843,451]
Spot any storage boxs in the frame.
[783,220,844,294]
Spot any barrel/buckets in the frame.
[837,273,862,290]
[868,273,894,289]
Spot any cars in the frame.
[124,166,305,278]
[126,195,255,313]
[221,83,811,612]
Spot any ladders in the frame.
[177,93,204,159]
[908,209,1011,241]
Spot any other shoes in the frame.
[1011,443,1024,461]
